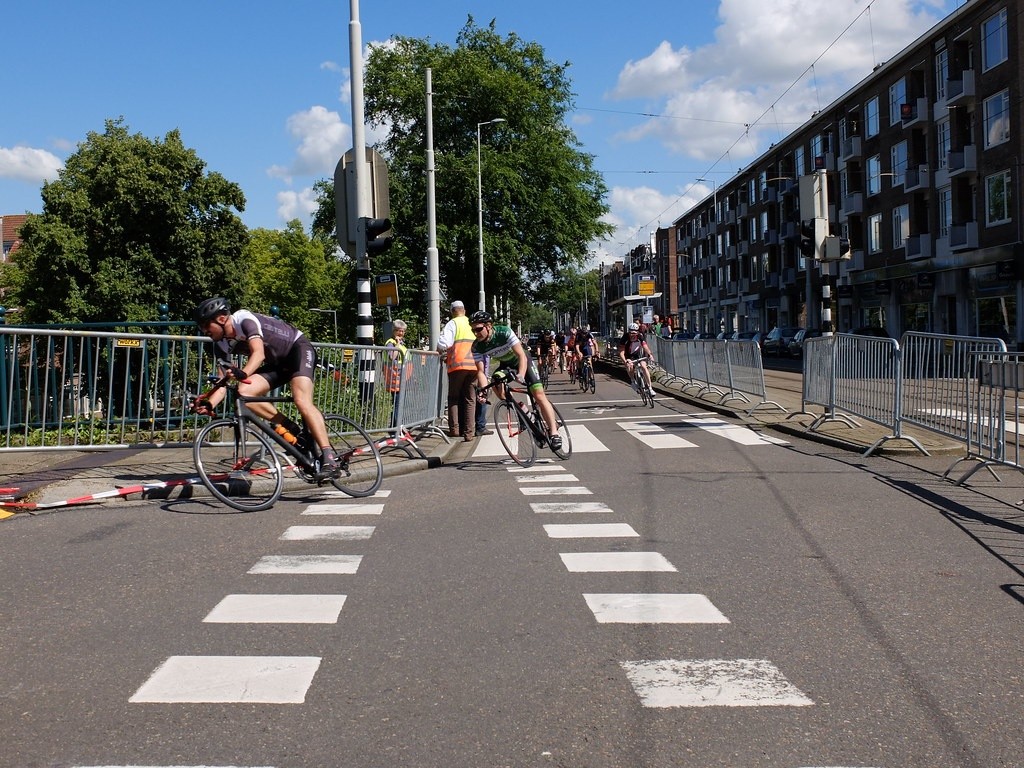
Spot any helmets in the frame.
[628,323,639,330]
[570,328,577,334]
[193,297,231,322]
[582,323,590,330]
[540,329,555,336]
[468,311,493,325]
[559,329,564,333]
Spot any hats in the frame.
[450,300,464,311]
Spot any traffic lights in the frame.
[799,218,815,257]
[825,236,850,261]
[355,216,390,257]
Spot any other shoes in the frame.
[631,379,635,386]
[549,431,562,450]
[563,365,566,371]
[447,431,459,437]
[464,437,473,441]
[650,389,656,397]
[314,463,342,482]
[475,429,494,436]
[516,411,527,432]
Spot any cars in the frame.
[662,325,893,377]
[527,336,540,360]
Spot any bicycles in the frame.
[472,370,575,468]
[190,358,384,513]
[628,354,655,409]
[576,355,598,394]
[537,350,575,386]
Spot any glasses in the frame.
[471,327,483,333]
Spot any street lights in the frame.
[696,178,719,330]
[478,118,502,318]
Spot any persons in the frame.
[471,311,562,452]
[384,319,414,437]
[420,300,674,442]
[191,297,342,480]
